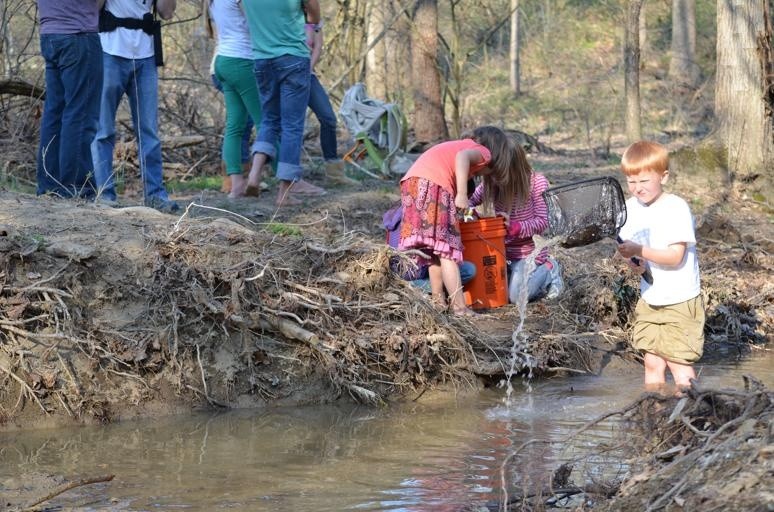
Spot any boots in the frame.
[326,160,359,185]
[220,162,268,194]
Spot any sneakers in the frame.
[543,259,564,298]
[36,188,115,202]
[144,197,177,214]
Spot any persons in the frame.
[202,0,362,206]
[36,0,179,215]
[614,140,706,386]
[398,125,512,317]
[469,135,566,306]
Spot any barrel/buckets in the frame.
[458,216,511,309]
